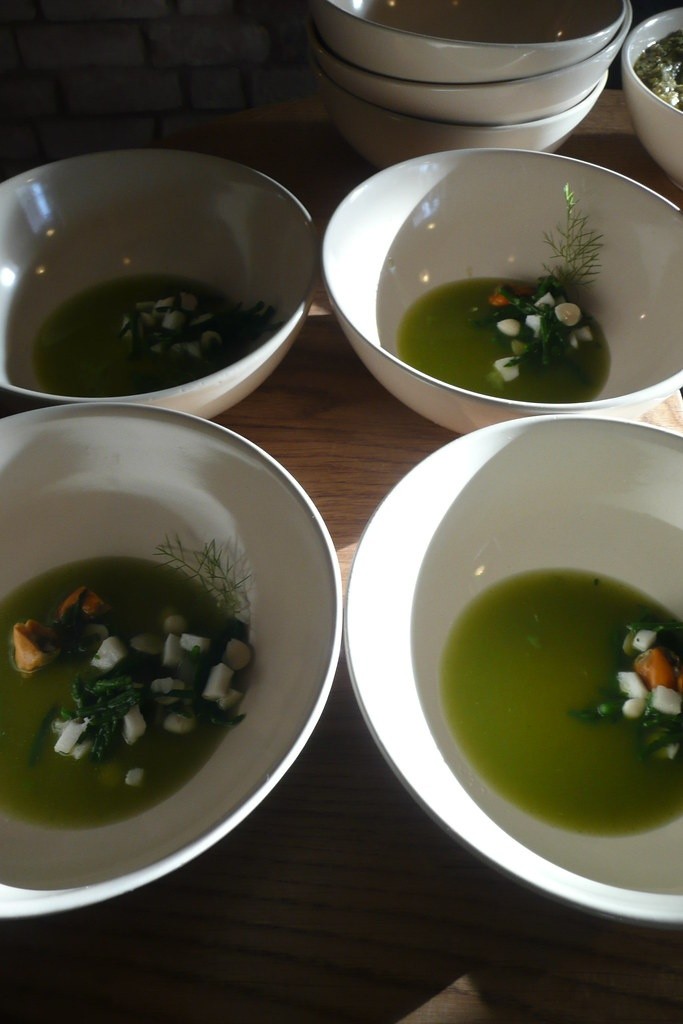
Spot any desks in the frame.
[0,88,683,1024]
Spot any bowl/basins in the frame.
[320,146,683,435]
[310,0,627,84]
[621,7,683,191]
[299,0,633,126]
[0,400,342,920]
[0,149,319,418]
[343,415,683,928]
[310,60,608,169]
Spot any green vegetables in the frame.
[121,288,286,379]
[491,181,604,370]
[31,594,249,761]
[568,618,682,777]
